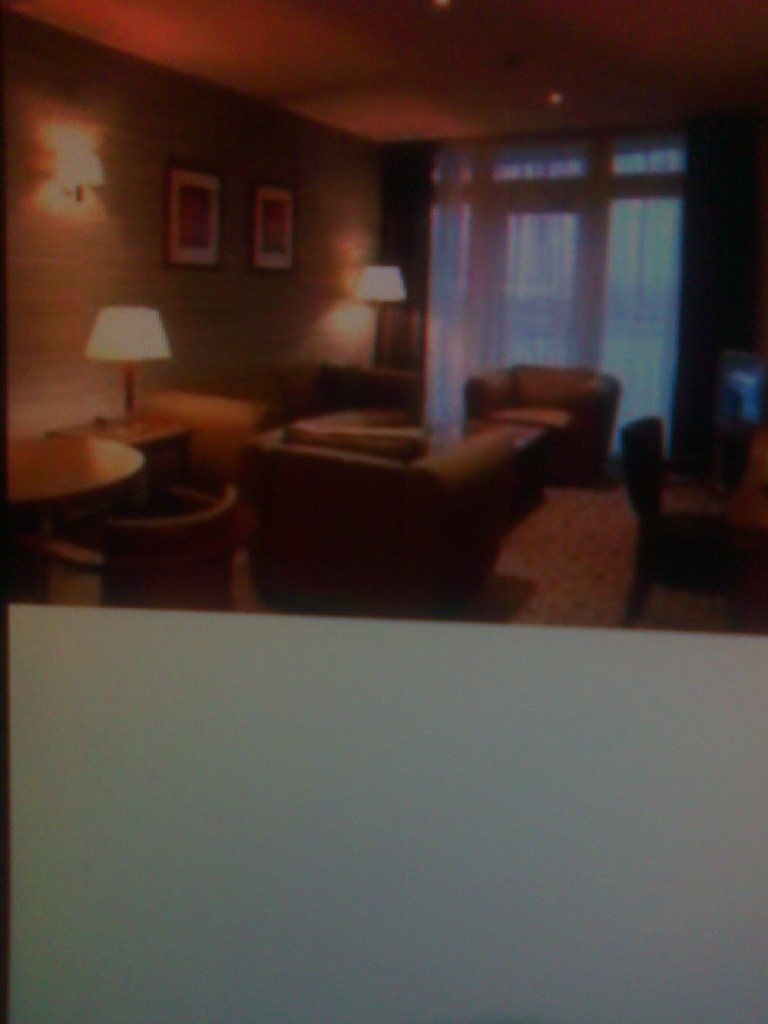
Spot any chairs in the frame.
[621,415,738,624]
[29,484,239,609]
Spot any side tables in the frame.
[46,423,193,483]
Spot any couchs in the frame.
[154,362,415,493]
[250,425,518,604]
[462,364,620,485]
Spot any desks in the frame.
[731,432,768,622]
[6,437,147,605]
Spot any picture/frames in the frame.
[250,182,295,274]
[164,159,226,271]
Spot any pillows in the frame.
[290,422,426,460]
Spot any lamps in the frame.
[85,306,173,426]
[354,265,409,363]
